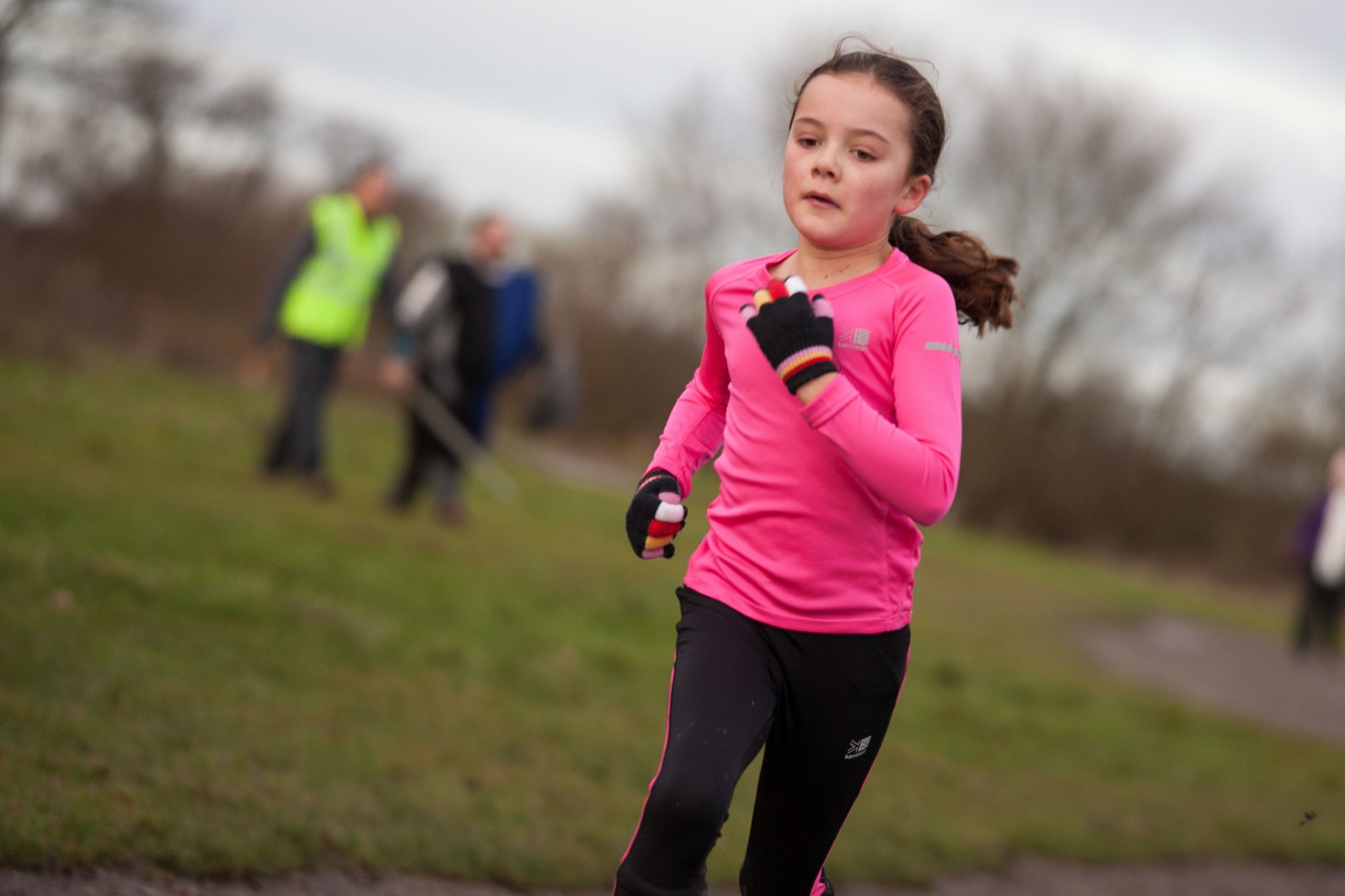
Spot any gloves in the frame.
[625,469,688,557]
[739,274,838,394]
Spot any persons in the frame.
[1288,451,1345,648]
[381,214,562,529]
[253,161,409,480]
[612,32,1018,896]
